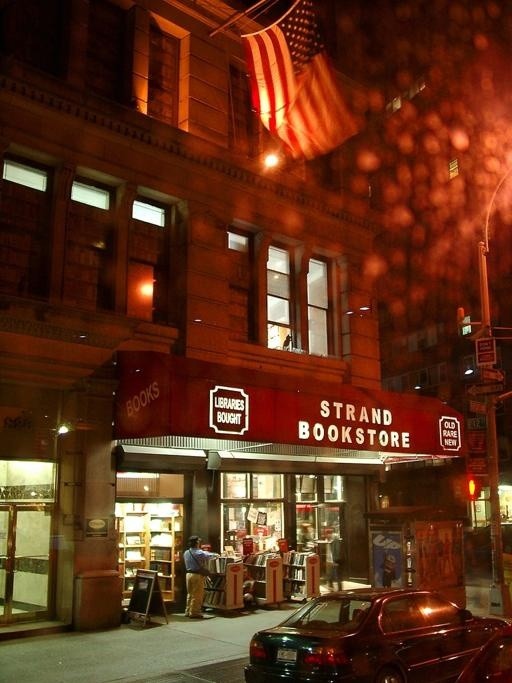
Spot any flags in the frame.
[240,0,359,162]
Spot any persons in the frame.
[326,533,347,592]
[382,551,395,589]
[183,536,218,618]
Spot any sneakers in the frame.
[185,613,203,618]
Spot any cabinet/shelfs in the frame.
[117,511,182,604]
[201,551,321,610]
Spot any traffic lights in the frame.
[447,472,480,503]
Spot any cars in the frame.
[241,583,512,682]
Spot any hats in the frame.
[189,536,202,544]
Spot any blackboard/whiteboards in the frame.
[127,570,155,615]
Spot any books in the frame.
[148,546,172,591]
[203,551,317,605]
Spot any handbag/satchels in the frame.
[198,566,209,576]
